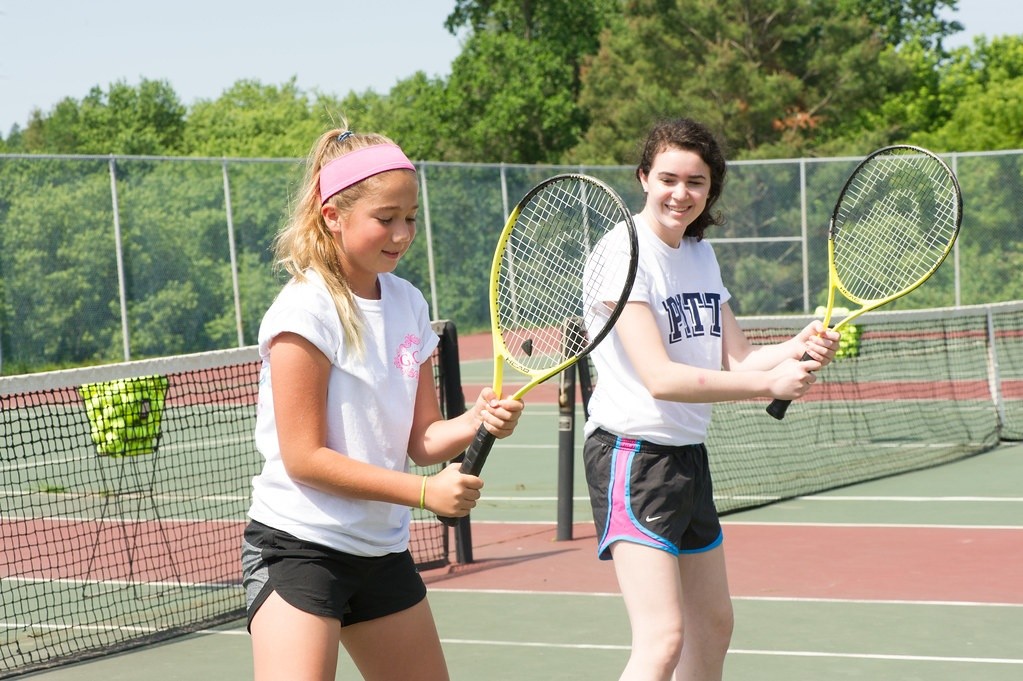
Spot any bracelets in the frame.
[419,474,427,512]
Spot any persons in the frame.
[238,126,526,681]
[580,119,839,680]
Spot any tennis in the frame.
[79,373,171,458]
[813,304,863,362]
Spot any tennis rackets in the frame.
[761,144,963,420]
[431,172,641,531]
